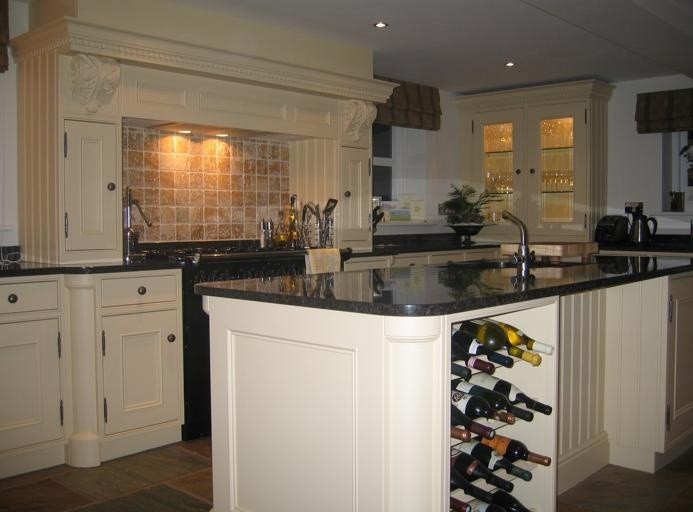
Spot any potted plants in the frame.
[442,183,492,245]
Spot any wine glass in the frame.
[483,120,573,192]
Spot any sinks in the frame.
[436,260,589,269]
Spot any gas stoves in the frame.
[152,245,303,280]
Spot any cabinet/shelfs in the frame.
[605,271,693,476]
[288,140,372,252]
[344,247,502,272]
[455,77,617,244]
[95,268,182,467]
[15,114,122,268]
[442,291,557,512]
[1,267,69,479]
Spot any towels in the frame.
[304,248,341,273]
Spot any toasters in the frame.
[593,214,626,247]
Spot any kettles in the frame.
[626,209,657,245]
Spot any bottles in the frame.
[446,315,555,511]
[257,195,301,249]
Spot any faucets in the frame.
[501,209,529,252]
[131,197,152,227]
